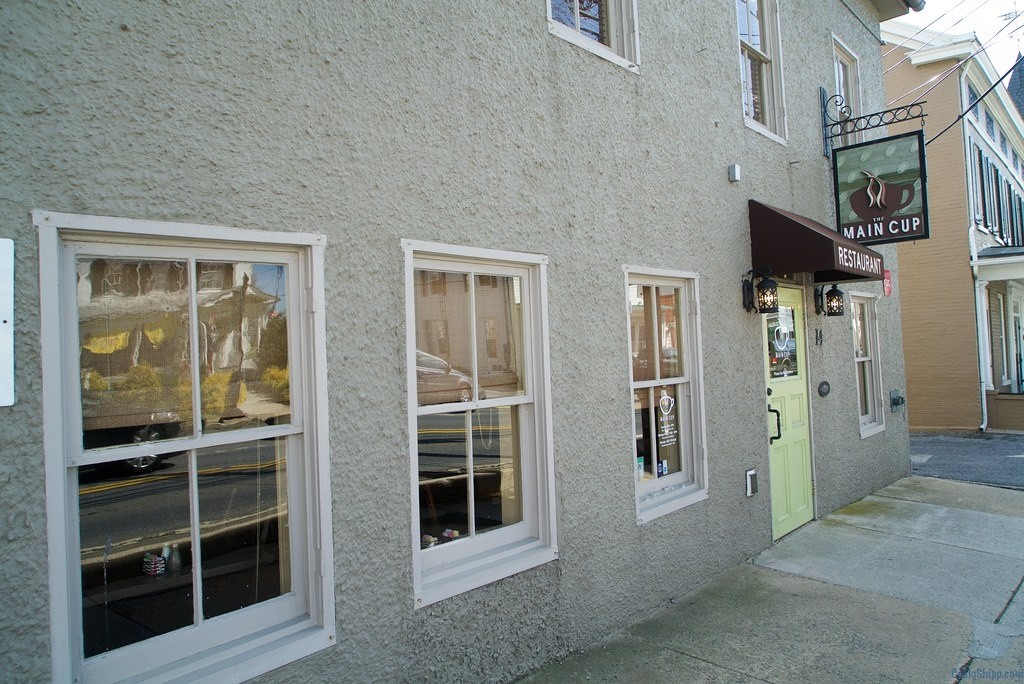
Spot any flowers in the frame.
[421,535,437,550]
[442,528,459,537]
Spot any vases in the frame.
[441,537,459,544]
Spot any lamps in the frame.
[814,284,844,316]
[741,265,779,313]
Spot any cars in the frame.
[770,339,797,377]
[82,403,204,473]
[416,350,480,413]
[633,349,679,378]
